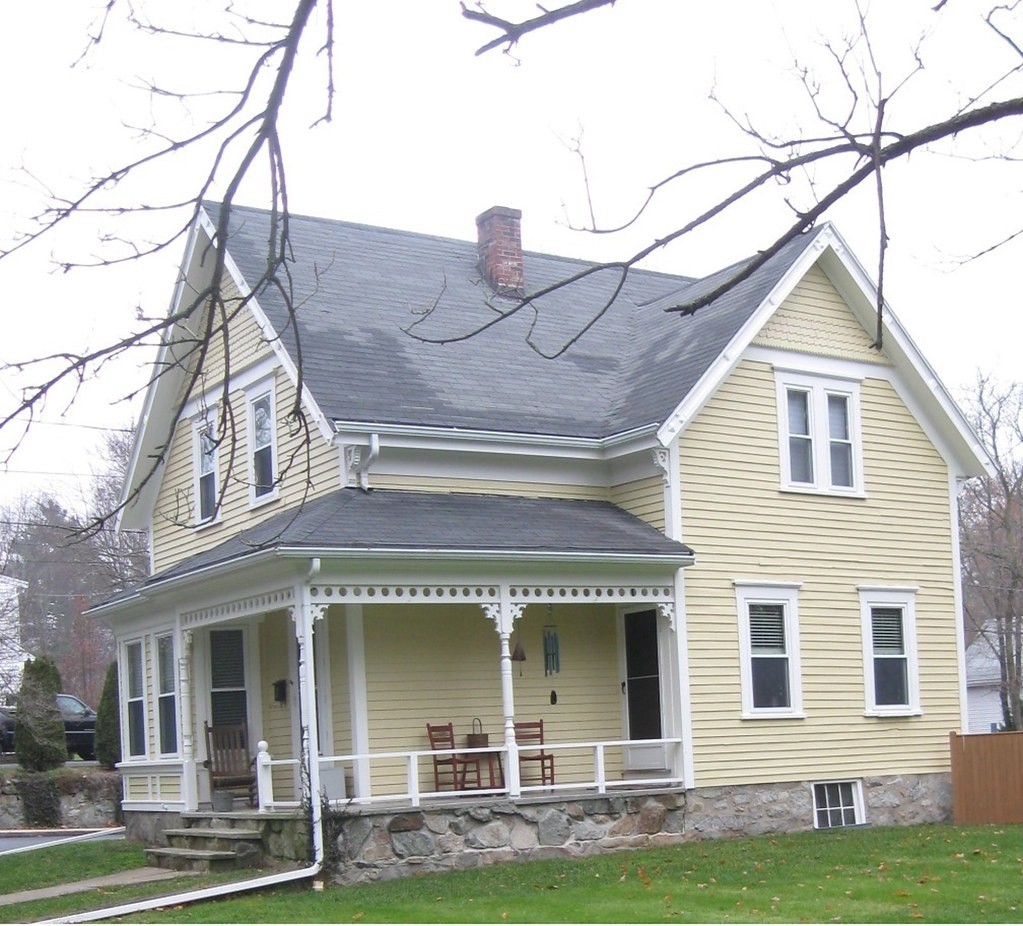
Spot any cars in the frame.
[0,693,98,758]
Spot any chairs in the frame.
[515,720,555,794]
[426,722,482,799]
[202,720,259,811]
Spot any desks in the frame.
[458,752,506,797]
